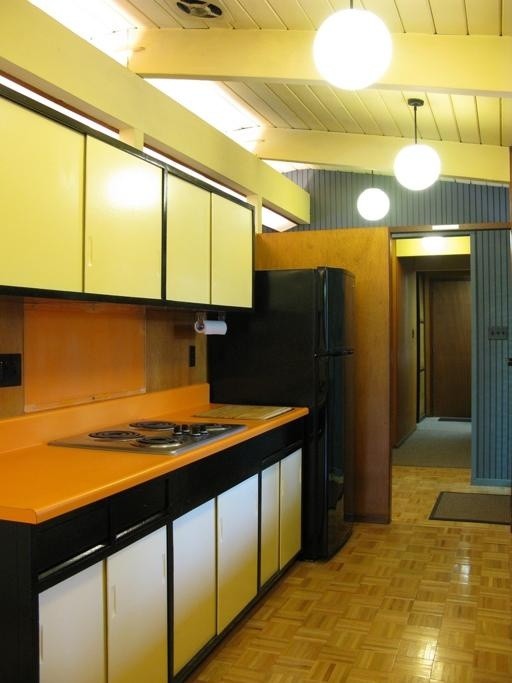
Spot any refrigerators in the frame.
[207,267,355,561]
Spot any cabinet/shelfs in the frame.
[0,82,165,310]
[0,477,172,682]
[165,160,256,316]
[259,411,312,598]
[170,433,260,683]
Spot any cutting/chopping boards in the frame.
[192,404,294,419]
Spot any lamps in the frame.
[393,97,442,192]
[356,169,390,222]
[312,0,394,92]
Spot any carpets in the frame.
[428,491,512,524]
[393,416,473,468]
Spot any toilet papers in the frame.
[194,320,228,336]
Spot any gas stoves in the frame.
[49,421,247,454]
